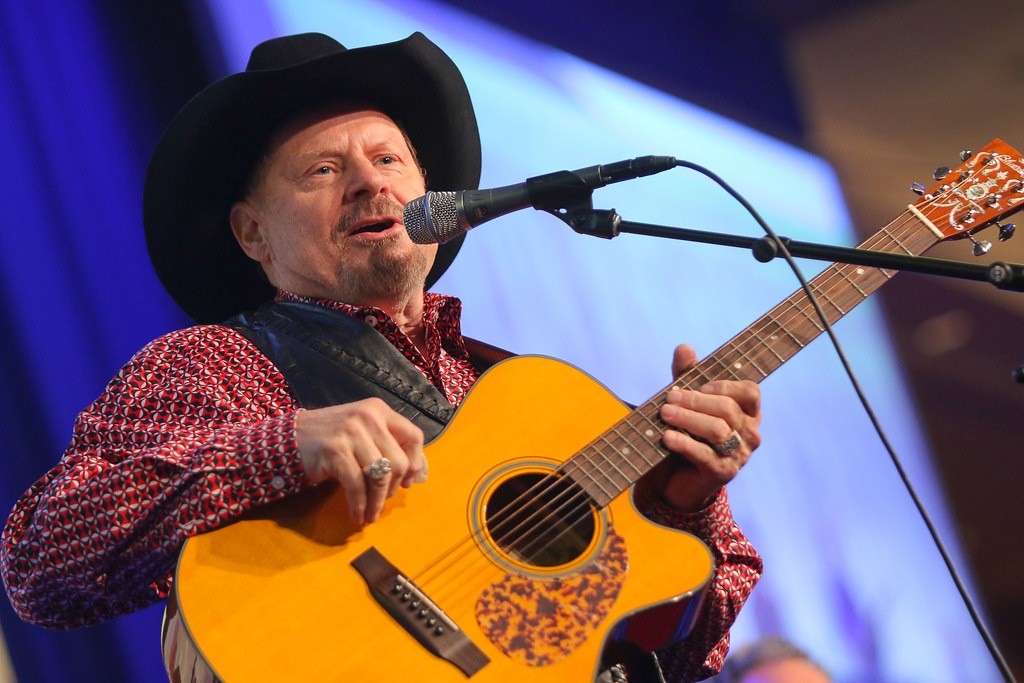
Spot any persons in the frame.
[1,29,763,682]
[715,639,831,683]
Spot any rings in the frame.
[362,457,390,480]
[717,429,740,455]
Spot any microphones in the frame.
[403,155,676,246]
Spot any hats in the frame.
[143,31,482,325]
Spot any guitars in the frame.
[163,139,1023,683]
